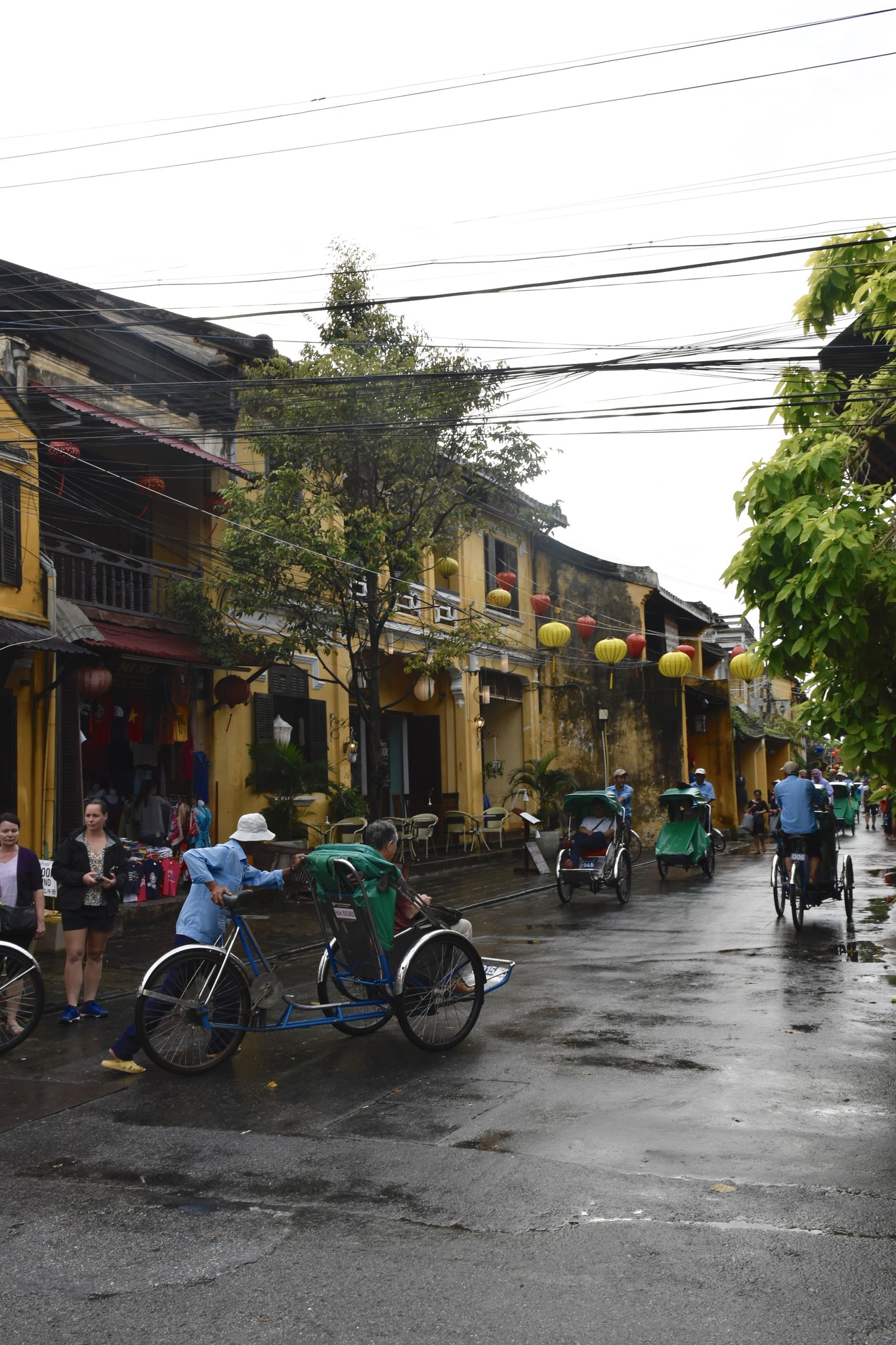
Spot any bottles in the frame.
[325,816,330,824]
[428,798,432,805]
[884,814,888,825]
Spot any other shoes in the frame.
[866,825,876,830]
[565,859,574,869]
[562,839,571,849]
[754,846,766,854]
[809,882,819,891]
[885,835,896,840]
[444,977,475,994]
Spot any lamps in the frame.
[512,789,529,810]
[356,666,370,695]
[412,674,435,702]
[345,727,359,763]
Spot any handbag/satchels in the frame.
[740,808,754,832]
[769,808,779,835]
[419,900,463,921]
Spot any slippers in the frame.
[206,1041,242,1058]
[101,1058,147,1073]
[6,1024,24,1035]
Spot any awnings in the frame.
[62,612,240,670]
[27,381,258,486]
[1,614,95,661]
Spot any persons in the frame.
[603,769,634,860]
[773,761,832,894]
[81,783,122,832]
[132,779,174,847]
[189,799,213,850]
[684,767,717,856]
[346,820,489,995]
[733,743,896,861]
[99,813,307,1074]
[662,797,703,862]
[0,813,48,1037]
[50,798,130,1025]
[557,799,619,889]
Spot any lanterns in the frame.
[202,491,233,541]
[45,433,81,496]
[66,663,113,734]
[409,543,778,710]
[135,471,165,520]
[213,675,251,733]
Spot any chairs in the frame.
[329,816,368,845]
[478,806,511,850]
[301,823,331,850]
[380,815,421,865]
[444,810,480,853]
[409,812,441,860]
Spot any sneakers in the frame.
[57,1005,80,1024]
[80,1001,109,1018]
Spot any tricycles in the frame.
[812,783,865,836]
[769,809,855,928]
[135,844,515,1074]
[655,786,725,878]
[555,792,641,905]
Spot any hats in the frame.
[228,813,275,842]
[694,768,706,775]
[613,769,628,778]
[780,761,800,775]
[862,779,867,782]
[837,771,848,777]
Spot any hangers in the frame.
[130,859,139,863]
[150,857,158,862]
[168,855,174,860]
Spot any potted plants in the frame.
[480,736,506,785]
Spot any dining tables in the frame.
[464,815,496,852]
[312,822,353,844]
[398,818,430,861]
